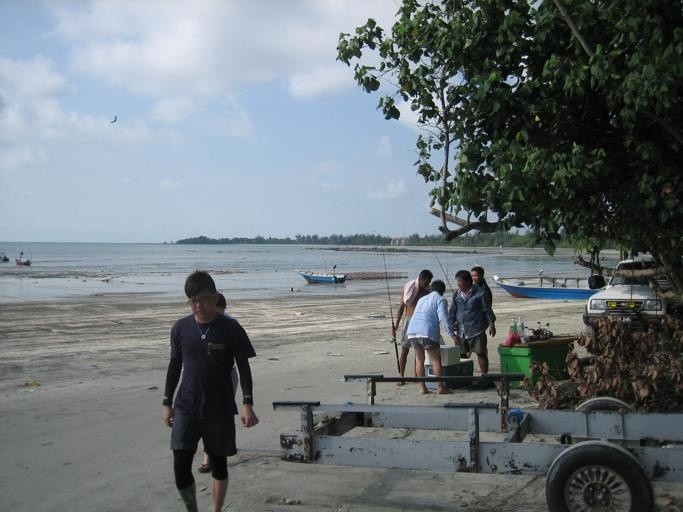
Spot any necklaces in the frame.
[193,318,213,341]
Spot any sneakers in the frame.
[198,462,209,473]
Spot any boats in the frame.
[297,271,346,283]
[496,276,607,299]
[15,258,31,265]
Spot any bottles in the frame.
[516,317,524,336]
[509,319,517,334]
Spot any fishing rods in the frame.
[424,233,455,297]
[381,241,401,374]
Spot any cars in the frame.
[583,259,681,332]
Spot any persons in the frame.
[448,269,496,377]
[407,280,456,394]
[196,292,239,474]
[460,266,493,372]
[390,269,435,388]
[161,270,260,512]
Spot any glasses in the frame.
[186,294,217,305]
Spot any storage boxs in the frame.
[498,345,568,389]
[424,358,474,390]
[424,345,462,367]
